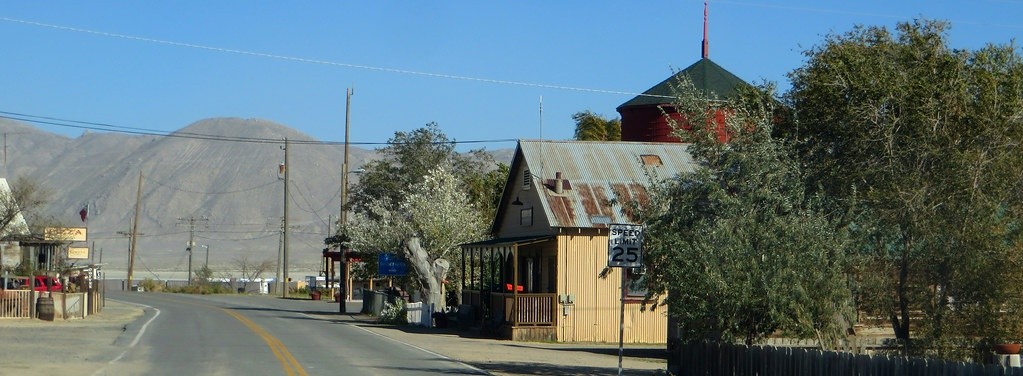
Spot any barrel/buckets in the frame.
[36,297,54,321]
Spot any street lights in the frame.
[276,138,293,299]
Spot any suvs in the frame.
[18,276,69,294]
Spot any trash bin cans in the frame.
[362,288,388,316]
